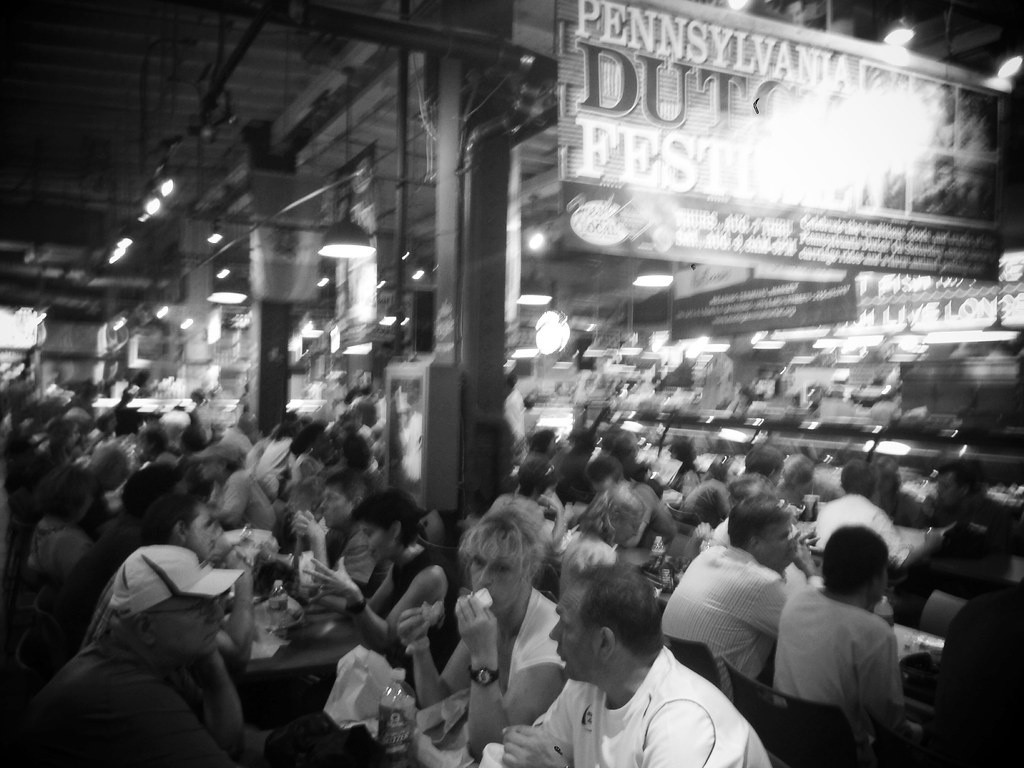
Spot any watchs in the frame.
[346,600,367,613]
[469,666,499,686]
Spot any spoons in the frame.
[294,606,332,618]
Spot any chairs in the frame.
[0,428,1024,767]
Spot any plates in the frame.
[254,595,305,630]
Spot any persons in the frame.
[502,564,771,768]
[773,524,932,768]
[1,356,1024,768]
[396,506,568,758]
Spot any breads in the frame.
[421,600,445,625]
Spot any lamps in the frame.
[205,184,248,304]
[315,62,375,259]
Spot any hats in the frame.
[109,543,246,621]
[193,443,240,463]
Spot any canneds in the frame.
[803,494,820,522]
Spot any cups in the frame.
[804,494,819,519]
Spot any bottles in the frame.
[378,668,416,768]
[238,522,255,548]
[649,536,667,562]
[874,595,894,630]
[268,580,289,640]
